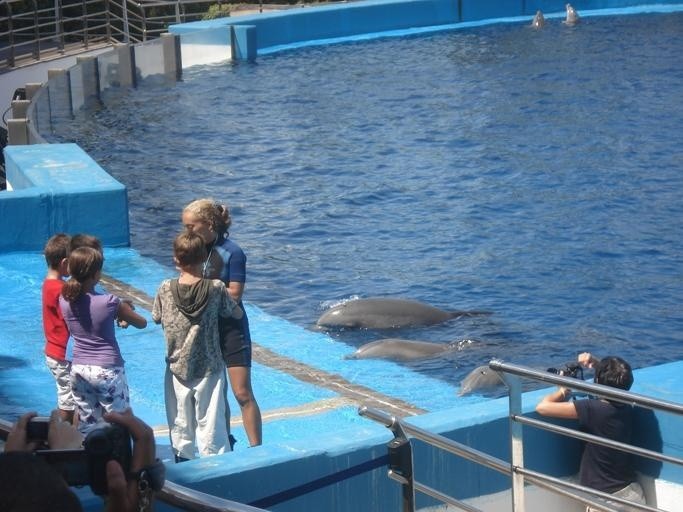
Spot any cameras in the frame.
[547,362,578,378]
[31,417,132,496]
[26,416,50,442]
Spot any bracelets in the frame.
[559,388,567,400]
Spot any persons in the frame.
[59,232,135,427]
[149,231,244,464]
[40,232,78,424]
[179,196,263,447]
[55,245,147,427]
[4,405,84,454]
[534,350,649,511]
[0,405,157,511]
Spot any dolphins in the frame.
[342,337,497,363]
[560,2,580,27]
[526,10,551,31]
[304,298,496,338]
[457,366,566,397]
